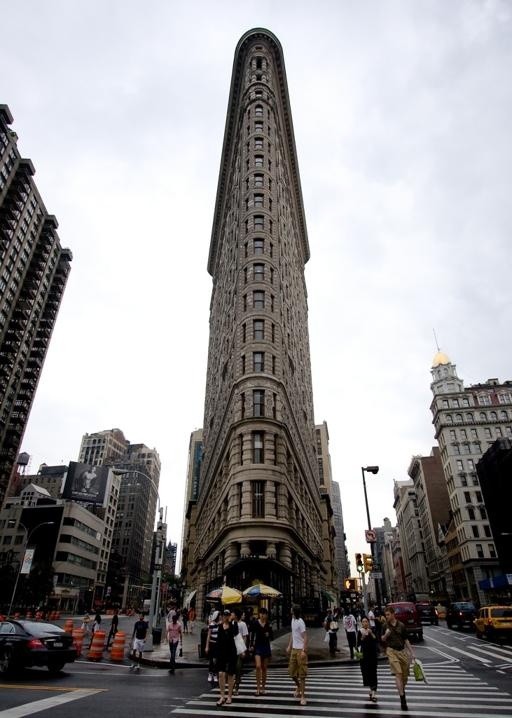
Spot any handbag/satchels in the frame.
[412,658,430,685]
[329,615,339,632]
[354,652,363,660]
[233,632,247,656]
[179,646,183,658]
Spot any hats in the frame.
[222,609,231,616]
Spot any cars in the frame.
[0,621,77,674]
[374,601,511,645]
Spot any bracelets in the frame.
[302,649,306,652]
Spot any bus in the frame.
[143,599,151,615]
[405,591,433,602]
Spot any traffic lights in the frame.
[345,579,356,590]
[363,553,373,572]
[356,553,362,572]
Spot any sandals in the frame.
[216,689,377,707]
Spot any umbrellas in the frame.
[242,584,283,601]
[205,585,242,609]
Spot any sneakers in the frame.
[207,673,212,682]
[399,692,409,711]
[213,674,219,683]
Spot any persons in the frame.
[130,613,148,669]
[357,616,379,702]
[286,605,308,704]
[165,607,274,704]
[80,610,90,632]
[380,607,414,711]
[86,610,101,649]
[105,609,119,650]
[324,604,379,658]
[78,466,98,493]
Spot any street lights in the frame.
[360,465,382,617]
[112,469,164,654]
[6,519,57,621]
